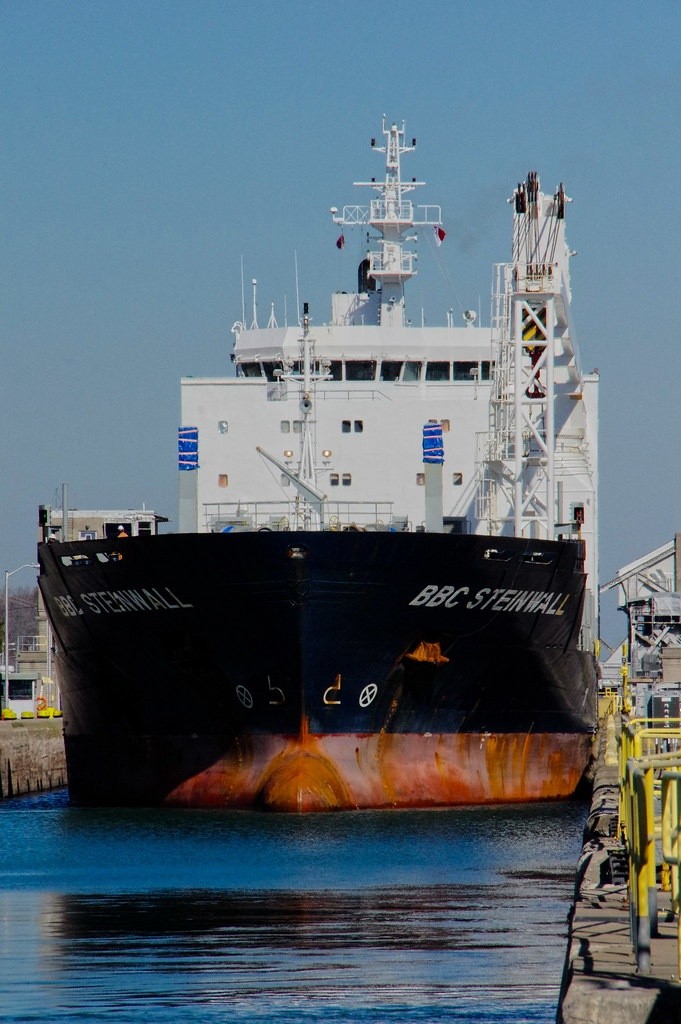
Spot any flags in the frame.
[432,225,448,247]
[336,235,345,250]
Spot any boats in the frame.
[33,108,604,815]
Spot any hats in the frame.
[118,525,124,531]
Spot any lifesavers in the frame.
[36,697,47,710]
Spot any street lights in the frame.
[6,562,41,707]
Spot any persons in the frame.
[116,525,129,560]
[589,368,601,377]
